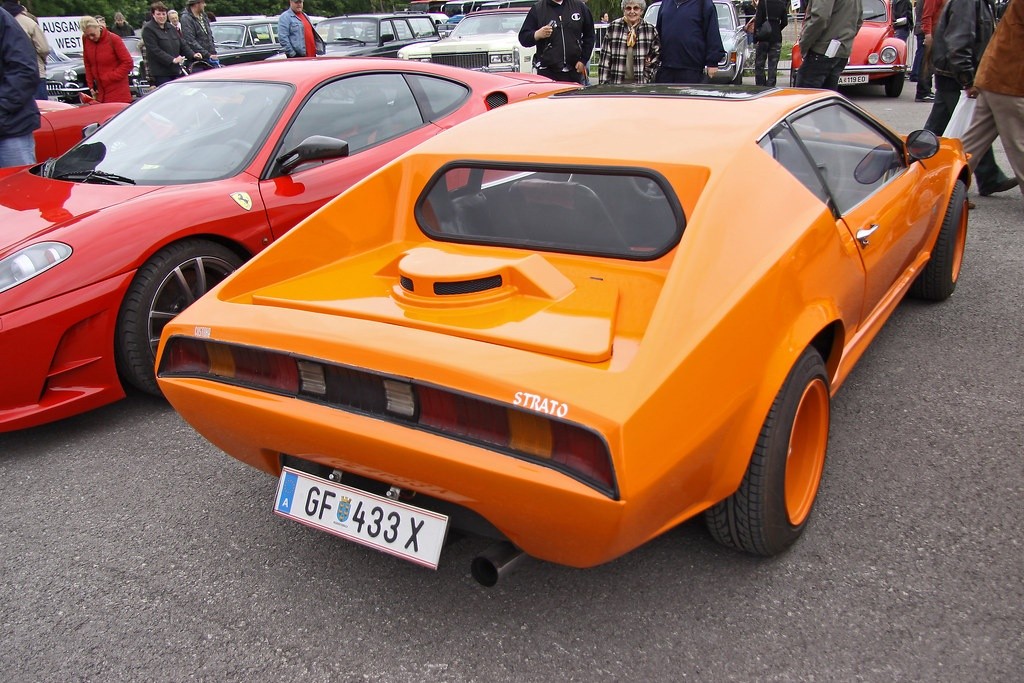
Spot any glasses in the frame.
[624,6,640,11]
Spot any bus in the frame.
[402,0,540,17]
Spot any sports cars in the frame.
[155,84,976,586]
[0,57,583,435]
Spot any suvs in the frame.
[265,11,440,59]
[396,7,544,76]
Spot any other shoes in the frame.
[981,177,1017,196]
[915,92,935,101]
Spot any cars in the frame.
[789,0,908,97]
[643,0,748,85]
[40,35,140,102]
[127,19,287,98]
[29,97,169,163]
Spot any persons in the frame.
[753,0,788,87]
[278,0,325,58]
[595,13,609,56]
[744,1,756,25]
[141,0,219,87]
[0,0,50,168]
[645,0,725,83]
[795,0,864,91]
[93,12,135,38]
[518,0,595,84]
[598,0,661,84]
[923,0,1024,209]
[79,15,134,103]
[891,0,950,102]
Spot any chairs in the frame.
[499,179,627,246]
[321,86,392,147]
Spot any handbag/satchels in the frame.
[759,21,772,39]
[531,52,551,72]
